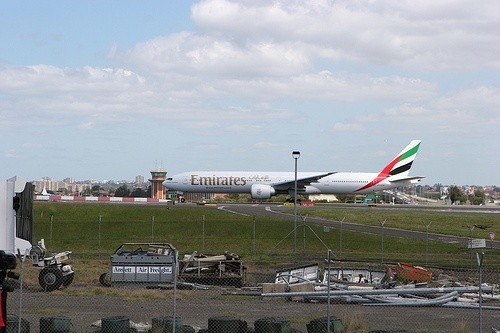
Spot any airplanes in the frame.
[162,139,426,203]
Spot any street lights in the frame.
[291,151,300,260]
